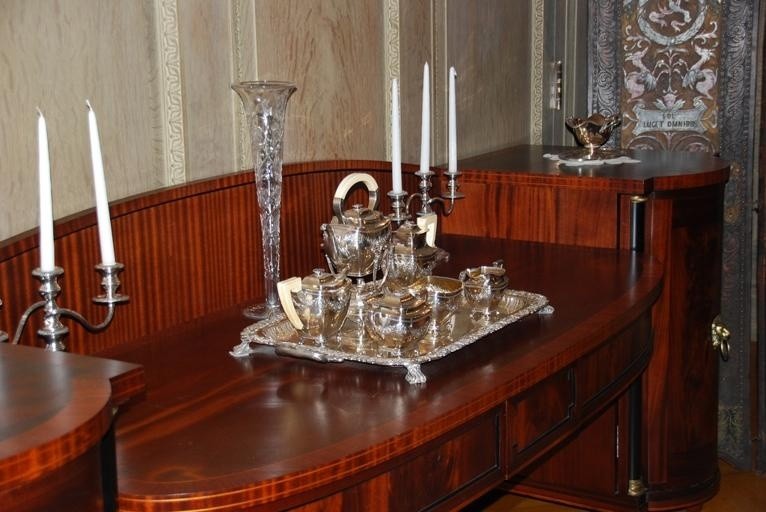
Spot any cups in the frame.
[461,257,508,318]
[356,283,431,355]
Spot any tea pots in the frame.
[320,172,396,292]
[383,221,440,293]
[278,267,355,345]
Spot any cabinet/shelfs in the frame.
[437,142,735,511]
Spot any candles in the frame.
[86,100,116,267]
[390,61,459,192]
[34,107,56,273]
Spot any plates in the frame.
[558,149,630,161]
[232,277,554,384]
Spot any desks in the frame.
[93,230,664,512]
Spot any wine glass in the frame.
[566,114,621,159]
